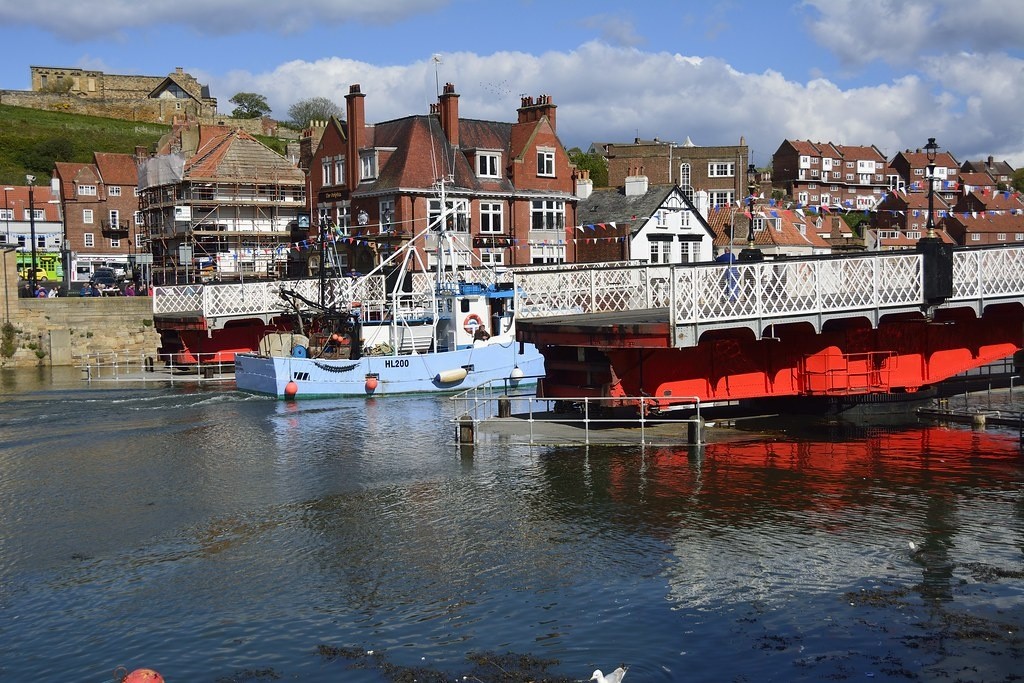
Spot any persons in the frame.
[22,283,62,298]
[80,280,154,297]
[473,325,490,342]
[715,247,739,303]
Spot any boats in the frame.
[233,115,548,402]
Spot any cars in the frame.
[87,268,122,299]
[18,267,49,282]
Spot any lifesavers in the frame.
[464,313,483,334]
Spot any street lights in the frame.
[922,137,941,238]
[744,164,758,250]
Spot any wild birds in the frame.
[590,663,630,683]
